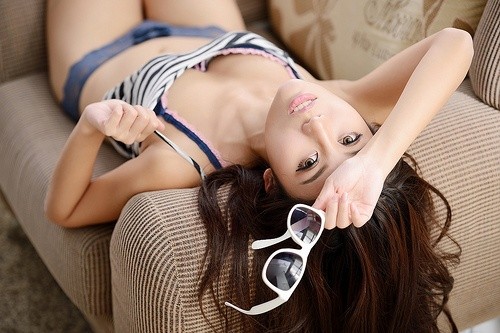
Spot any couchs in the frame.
[0,0,500,333]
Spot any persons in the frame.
[48,0,474,333]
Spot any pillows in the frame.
[265,0,488,82]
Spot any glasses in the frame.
[224,203,327,314]
